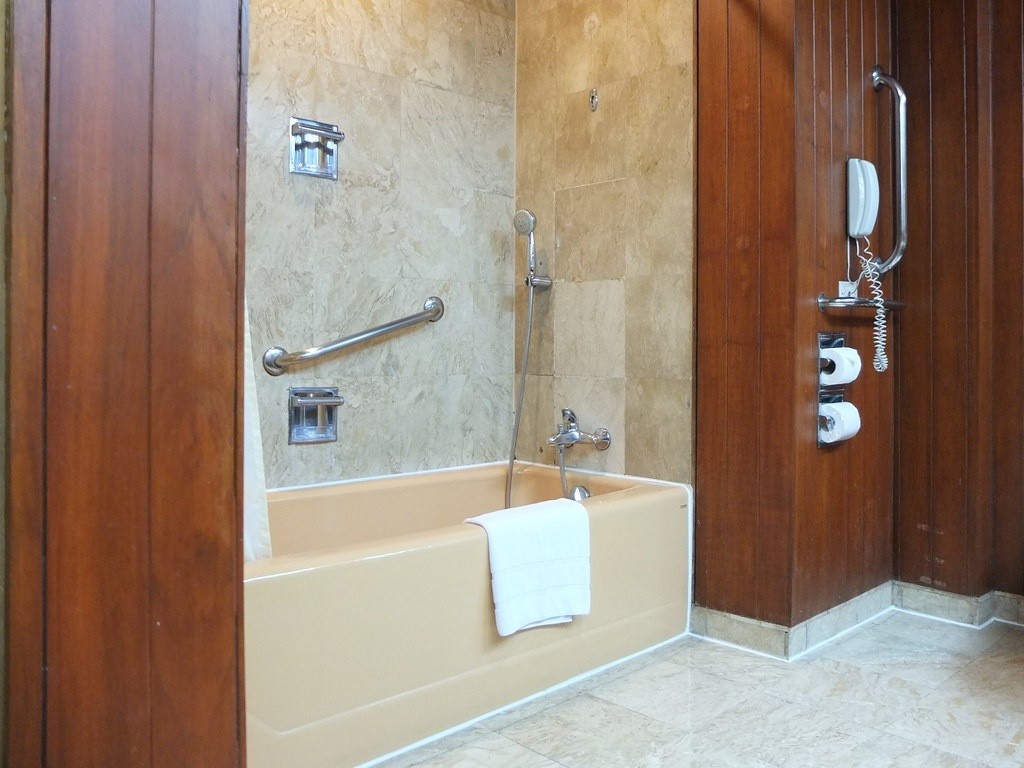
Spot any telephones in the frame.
[846,158,880,239]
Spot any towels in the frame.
[463,498,592,638]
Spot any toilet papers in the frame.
[816,347,862,444]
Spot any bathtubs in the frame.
[237,457,696,768]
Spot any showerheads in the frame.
[512,208,539,289]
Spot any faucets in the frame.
[545,408,580,448]
